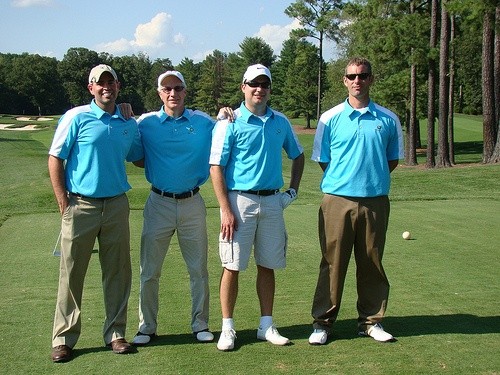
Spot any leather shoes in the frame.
[106,338,133,354]
[51,345,72,362]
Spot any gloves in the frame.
[280,188,299,210]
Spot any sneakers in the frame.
[217,327,241,352]
[358,322,394,342]
[308,329,328,345]
[257,323,290,345]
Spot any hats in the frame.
[89,65,119,85]
[243,64,271,84]
[158,71,184,86]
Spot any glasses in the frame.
[345,73,371,81]
[244,80,271,89]
[160,86,184,93]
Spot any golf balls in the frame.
[402,231,410,240]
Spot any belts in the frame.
[151,185,200,199]
[228,189,281,197]
[71,191,116,200]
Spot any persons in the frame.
[208,64,306,351]
[48,64,146,363]
[117,71,237,347]
[308,57,406,346]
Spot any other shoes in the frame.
[194,328,215,342]
[130,332,157,344]
